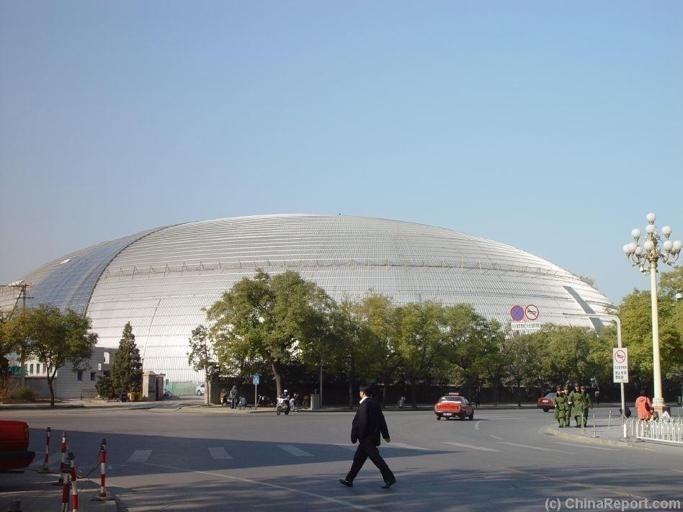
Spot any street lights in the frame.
[623,213,681,420]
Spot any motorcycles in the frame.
[276,397,291,415]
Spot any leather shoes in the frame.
[338,479,353,487]
[381,479,396,488]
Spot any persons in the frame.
[289,397,295,412]
[594,389,600,406]
[634,388,656,420]
[279,388,289,400]
[336,383,399,490]
[238,394,247,410]
[229,385,238,410]
[219,389,225,406]
[554,382,592,428]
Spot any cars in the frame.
[538,393,557,411]
[435,393,474,421]
[196,384,205,396]
[0,421,35,475]
